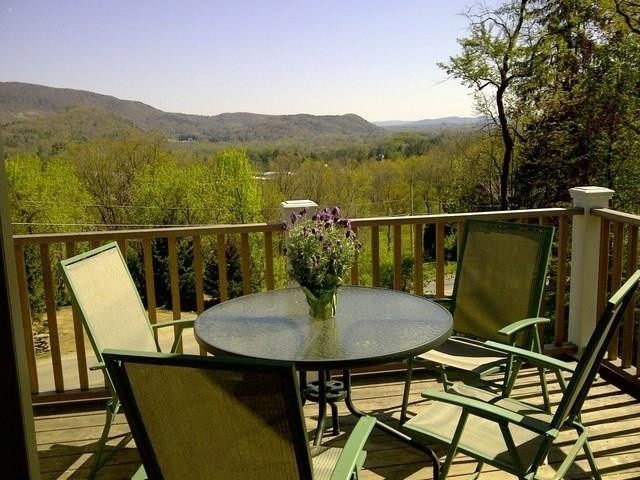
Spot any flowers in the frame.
[277,205,364,288]
